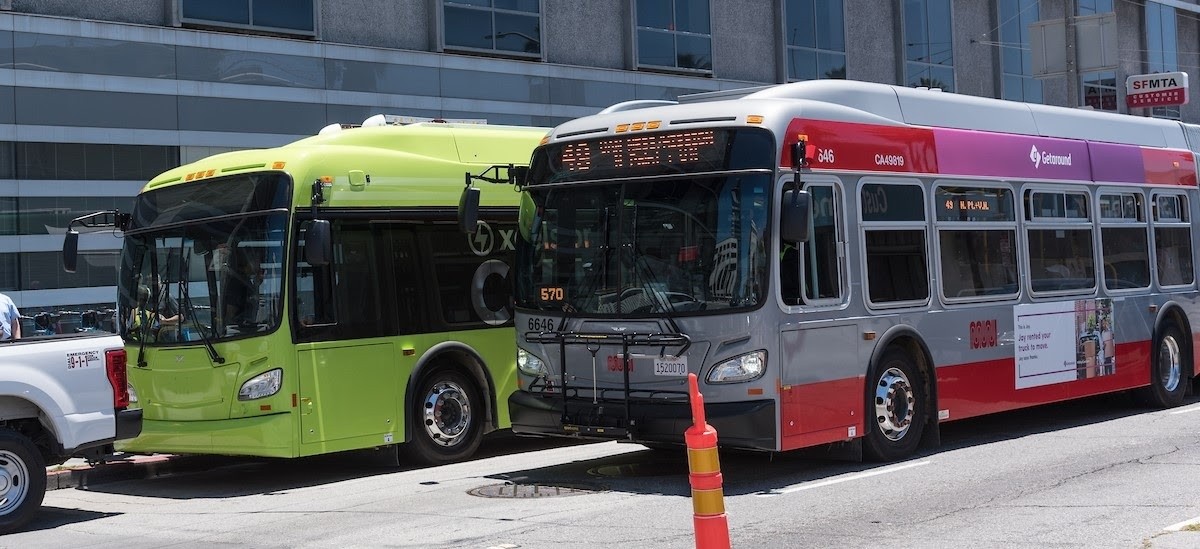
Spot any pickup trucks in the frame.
[0,330,144,538]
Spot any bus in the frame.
[63,113,636,471]
[458,78,1200,466]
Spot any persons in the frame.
[779,240,812,306]
[126,274,186,343]
[1100,319,1113,375]
[632,246,683,288]
[0,292,21,341]
[224,249,264,328]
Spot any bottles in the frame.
[182,327,191,342]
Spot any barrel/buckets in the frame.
[59,319,81,334]
[22,320,35,336]
